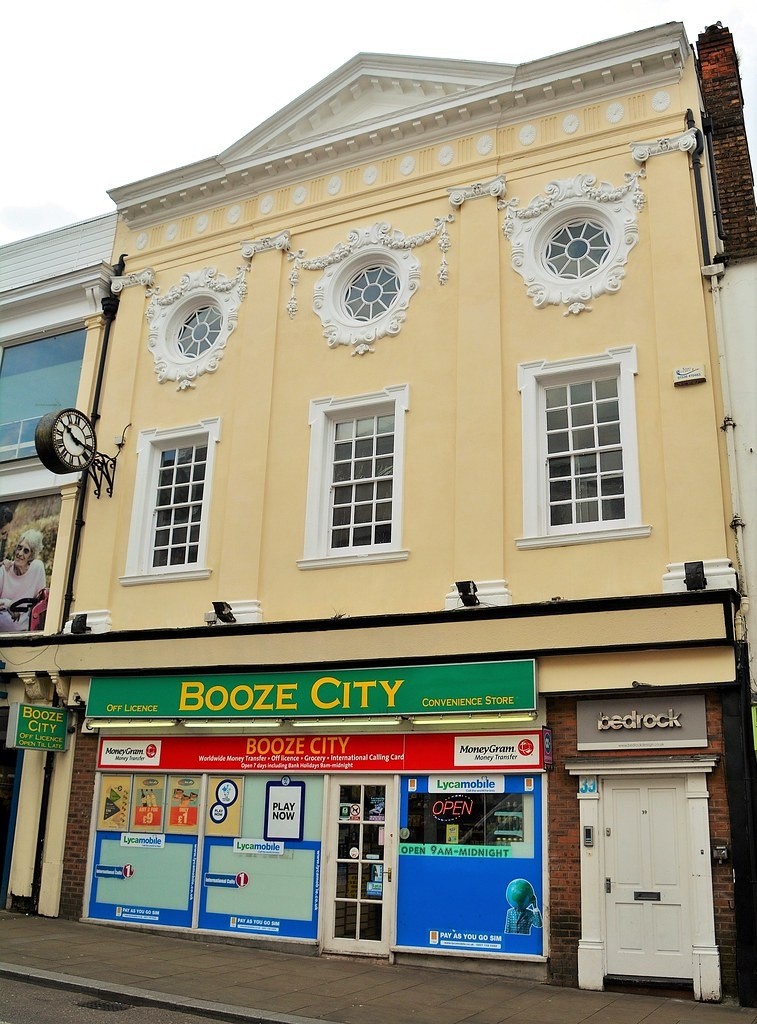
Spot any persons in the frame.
[0,506,46,632]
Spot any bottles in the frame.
[496,816,522,830]
[338,836,347,858]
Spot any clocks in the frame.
[35,407,117,499]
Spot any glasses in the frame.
[18,544,32,554]
[0,530,8,538]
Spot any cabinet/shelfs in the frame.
[494,812,523,838]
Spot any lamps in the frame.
[683,561,707,591]
[71,614,91,635]
[455,581,480,606]
[212,602,237,623]
